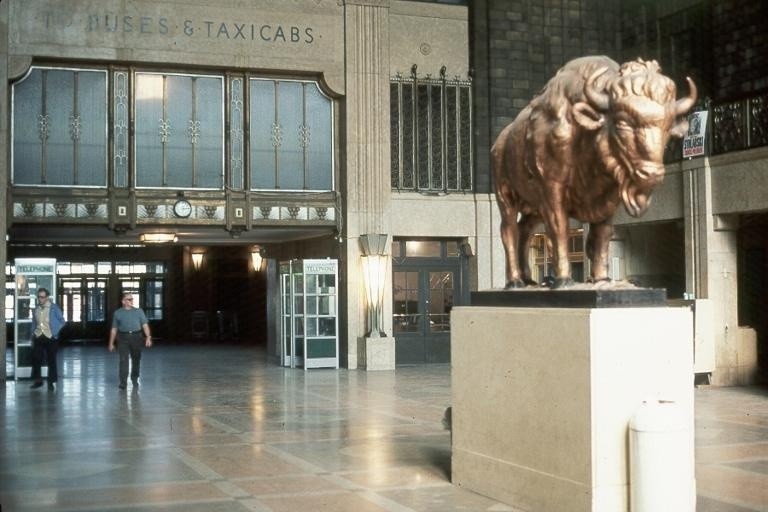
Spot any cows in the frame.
[487,54,698,290]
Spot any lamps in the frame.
[359,234,388,338]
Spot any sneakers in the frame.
[119,378,138,389]
[31,382,53,390]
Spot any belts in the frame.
[121,330,144,334]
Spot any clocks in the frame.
[173,199,192,218]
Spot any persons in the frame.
[28,288,65,391]
[108,293,153,389]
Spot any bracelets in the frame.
[146,335,151,337]
